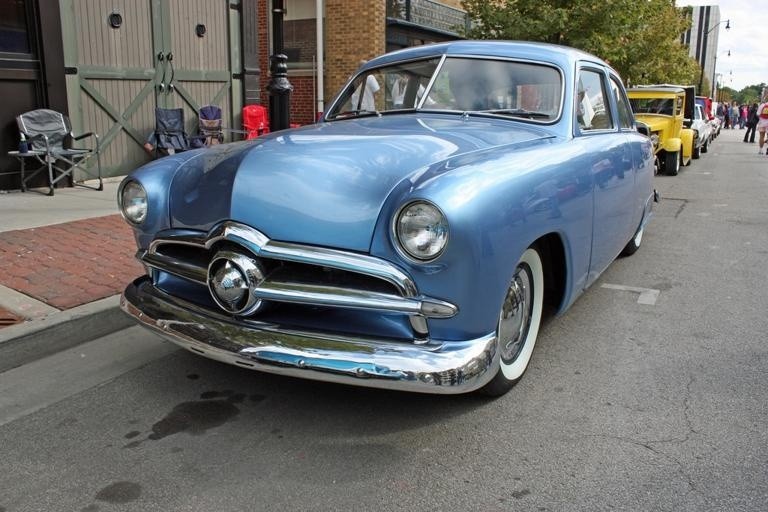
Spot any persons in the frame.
[349,60,382,112]
[391,73,439,108]
[717,95,768,154]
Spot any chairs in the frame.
[8,107,105,198]
[143,104,272,161]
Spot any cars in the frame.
[624,84,721,176]
[117,38,656,403]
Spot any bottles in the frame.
[19,139,28,152]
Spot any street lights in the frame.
[698,19,732,102]
